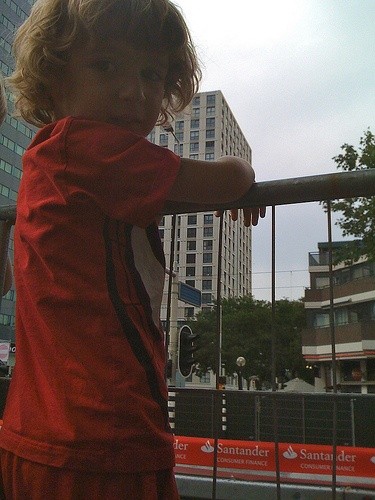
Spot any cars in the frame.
[0,360,9,378]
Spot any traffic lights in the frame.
[181,332,203,365]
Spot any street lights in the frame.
[237,357,246,391]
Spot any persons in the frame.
[1,0,266,500]
